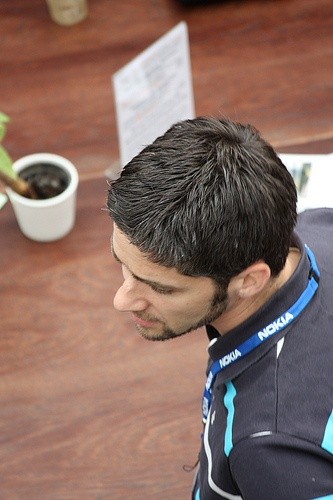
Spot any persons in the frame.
[104,114,333,500]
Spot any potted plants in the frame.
[0,111,79,242]
